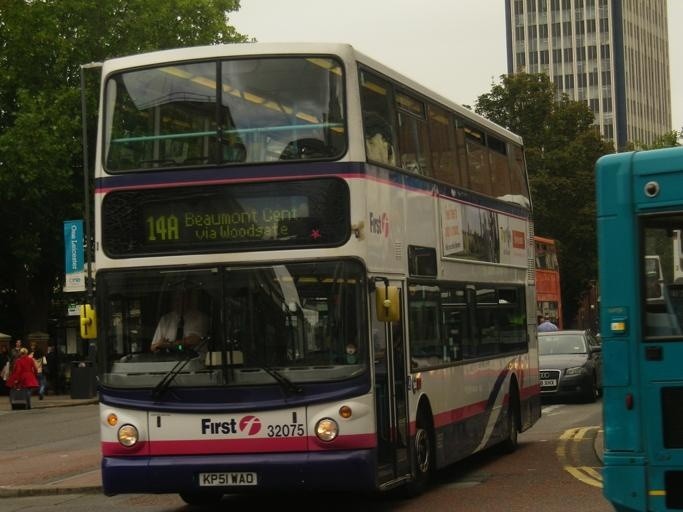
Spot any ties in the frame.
[176,315,183,339]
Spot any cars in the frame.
[58,360,101,396]
[537,329,601,404]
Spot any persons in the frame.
[538,315,544,325]
[150,292,211,365]
[6,338,60,400]
[537,314,558,332]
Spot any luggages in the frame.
[10,383,31,410]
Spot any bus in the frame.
[594,148,681,512]
[576,283,601,329]
[533,235,563,331]
[79,42,542,512]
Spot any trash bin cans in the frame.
[70,360,97,399]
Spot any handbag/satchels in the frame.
[42,367,51,375]
